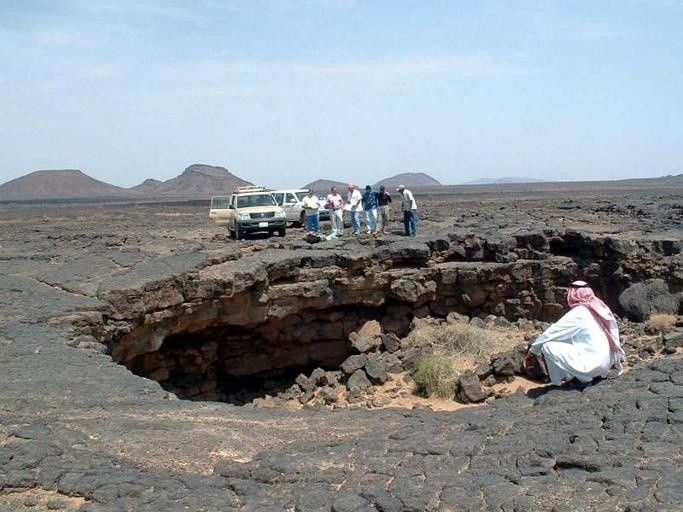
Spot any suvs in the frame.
[208,184,329,239]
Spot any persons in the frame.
[301,191,321,233]
[325,186,344,236]
[347,184,392,234]
[523,280,620,387]
[395,185,417,236]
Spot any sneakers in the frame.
[404,233,417,237]
[335,230,389,237]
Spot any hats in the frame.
[396,184,405,191]
[365,185,371,190]
[380,186,385,190]
